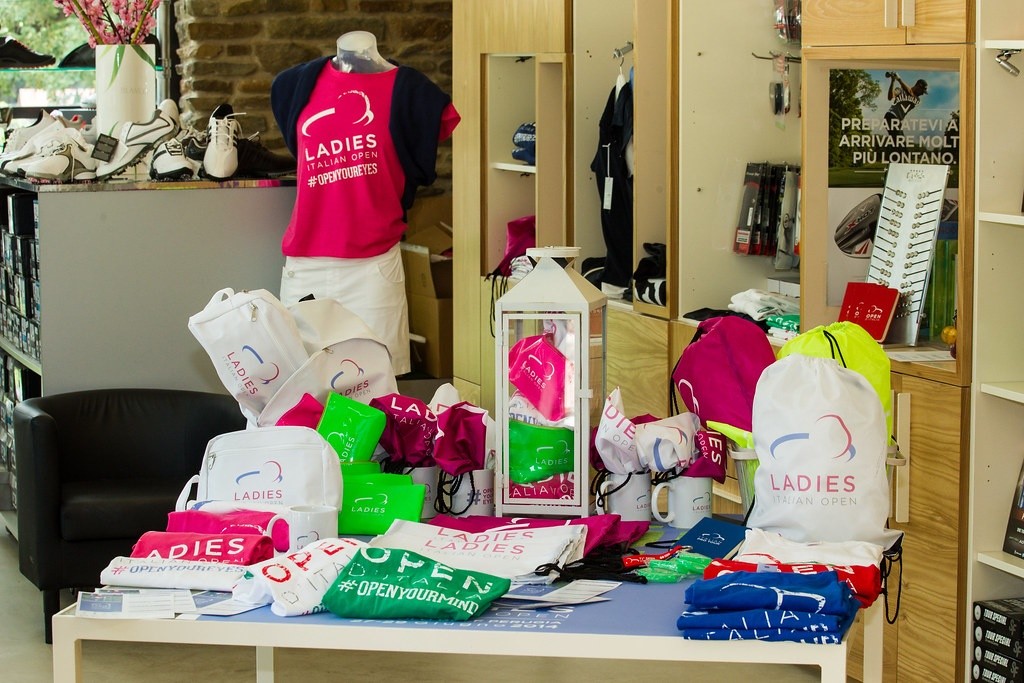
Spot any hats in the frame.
[917,79,928,94]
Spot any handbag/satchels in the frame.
[100,287,905,643]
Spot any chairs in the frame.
[12,386,247,645]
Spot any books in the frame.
[838,282,901,343]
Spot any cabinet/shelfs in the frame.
[0,183,297,544]
[965,0,1024,683]
[447,0,976,683]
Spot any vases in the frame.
[94,43,156,140]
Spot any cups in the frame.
[731,440,760,520]
[596,474,652,521]
[402,464,439,518]
[266,505,338,558]
[651,475,712,529]
[450,470,494,519]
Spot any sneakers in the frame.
[0,98,299,184]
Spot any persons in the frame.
[270,30,462,376]
[884,71,928,163]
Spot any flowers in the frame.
[52,0,163,88]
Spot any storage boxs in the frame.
[399,223,453,379]
[971,597,1024,683]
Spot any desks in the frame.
[49,527,904,683]
